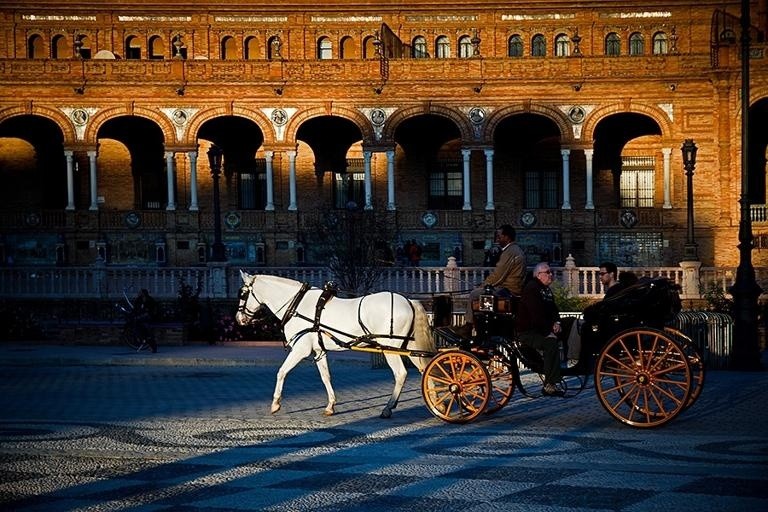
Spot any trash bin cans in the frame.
[408,294,454,330]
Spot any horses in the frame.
[234,268,446,419]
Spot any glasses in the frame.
[598,272,607,275]
[540,270,551,274]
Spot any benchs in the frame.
[37,297,210,347]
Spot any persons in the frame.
[619,272,639,287]
[406,239,419,278]
[521,262,576,396]
[560,262,626,369]
[465,225,530,337]
[131,289,161,354]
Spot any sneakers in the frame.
[447,326,472,340]
[542,383,565,396]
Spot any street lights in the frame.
[680,138,698,261]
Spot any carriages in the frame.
[234,267,707,428]
